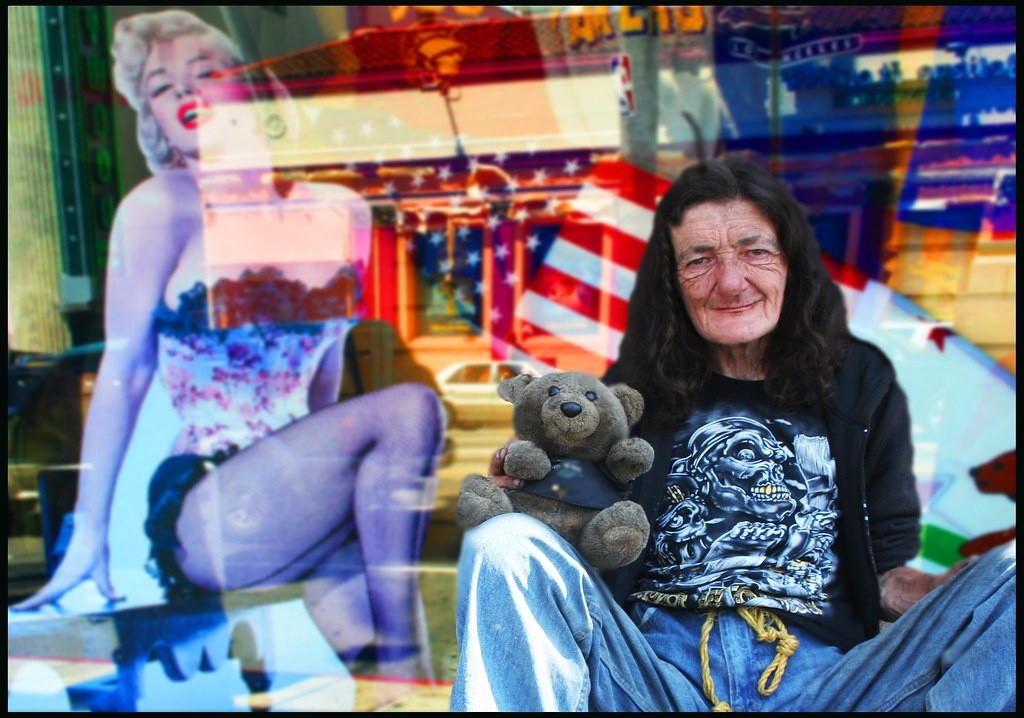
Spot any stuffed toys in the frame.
[458,370,656,570]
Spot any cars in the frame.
[1,340,112,540]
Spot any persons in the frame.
[11,10,446,702]
[450,152,1018,714]
